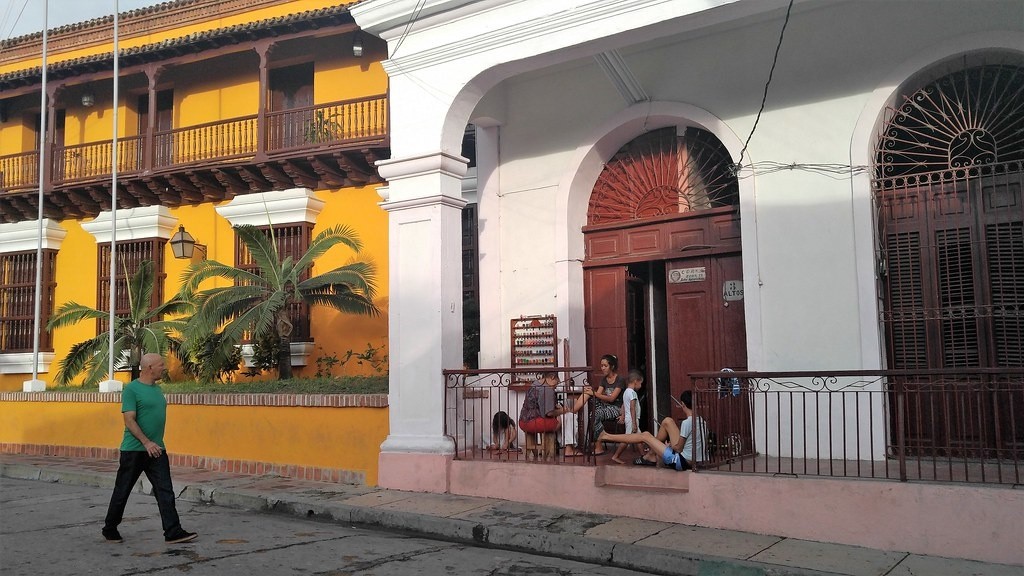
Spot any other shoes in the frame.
[507,447,522,452]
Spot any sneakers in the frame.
[101,528,123,543]
[165,528,198,544]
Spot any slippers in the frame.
[593,418,604,442]
[633,456,657,466]
[565,451,583,456]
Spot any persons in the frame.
[492,411,518,453]
[518,371,583,457]
[102,352,198,545]
[568,353,626,456]
[612,369,648,464]
[593,390,710,471]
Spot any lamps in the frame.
[82,83,95,107]
[170,223,206,262]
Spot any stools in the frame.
[525,430,556,460]
